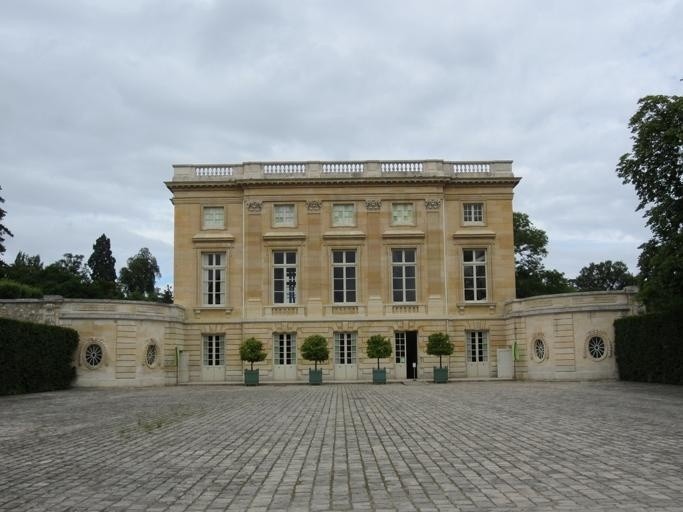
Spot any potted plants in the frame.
[366,334,393,385]
[239,337,267,386]
[425,332,454,384]
[300,335,329,385]
